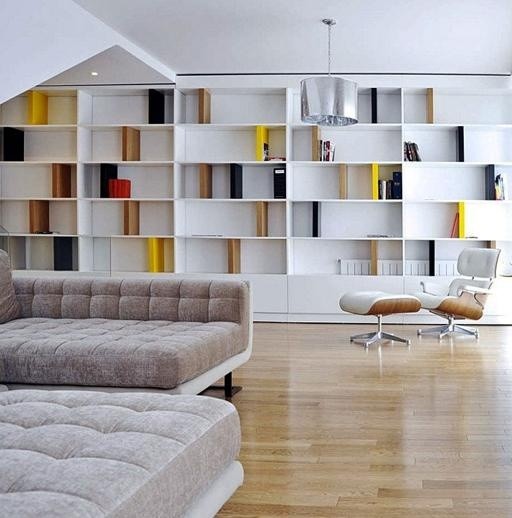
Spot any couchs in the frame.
[1,251,254,399]
[0,390,244,518]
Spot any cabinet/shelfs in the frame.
[0,84,511,323]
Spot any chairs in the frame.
[417,246,501,341]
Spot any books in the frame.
[378,172,402,199]
[318,139,335,161]
[494,174,508,200]
[450,212,459,238]
[269,156,286,161]
[274,167,286,198]
[404,141,421,161]
[367,234,393,238]
[108,179,131,197]
[264,143,269,161]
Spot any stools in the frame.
[339,288,420,353]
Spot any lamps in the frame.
[298,17,360,128]
